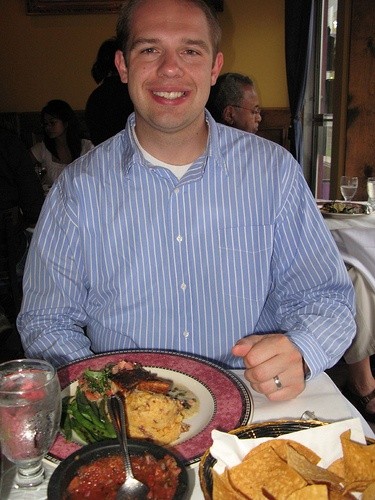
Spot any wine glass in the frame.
[0,359,62,500]
[339,176,359,201]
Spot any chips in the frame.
[210,429,375,500]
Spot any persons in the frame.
[0,116,46,323]
[30,36,261,197]
[17,0,358,403]
[327,26,335,71]
[336,263,375,419]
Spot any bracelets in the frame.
[302,358,311,381]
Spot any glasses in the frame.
[230,105,261,118]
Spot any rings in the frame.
[273,375,281,388]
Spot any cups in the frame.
[35,164,46,180]
[367,177,375,212]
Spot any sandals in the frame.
[341,378,375,422]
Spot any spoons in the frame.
[106,394,149,500]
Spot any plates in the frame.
[317,204,372,218]
[44,347,251,465]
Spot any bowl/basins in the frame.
[47,438,188,500]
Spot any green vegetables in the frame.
[61,367,119,445]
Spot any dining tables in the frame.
[1,369,375,500]
[320,213,375,294]
